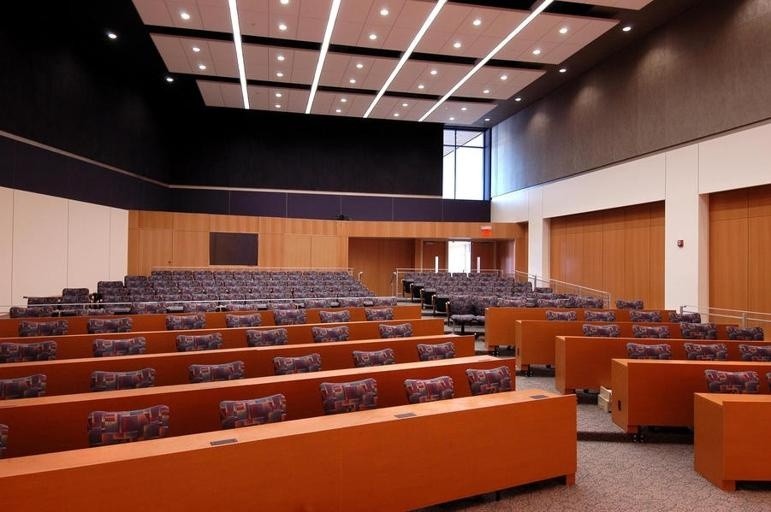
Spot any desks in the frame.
[0,387,580,510]
[552,334,769,394]
[605,354,769,435]
[513,319,742,374]
[0,332,483,396]
[689,387,770,495]
[480,306,678,355]
[0,349,521,453]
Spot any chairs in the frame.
[627,309,663,323]
[580,323,622,337]
[623,341,675,361]
[682,341,732,362]
[350,347,398,369]
[1,271,611,337]
[0,372,50,402]
[415,340,460,361]
[681,320,716,339]
[86,401,173,447]
[187,360,249,383]
[725,322,764,339]
[614,298,646,310]
[629,323,671,338]
[318,377,380,413]
[737,340,770,363]
[703,367,761,394]
[89,366,157,392]
[403,374,459,405]
[214,391,291,429]
[669,310,702,324]
[271,351,324,374]
[464,364,515,395]
